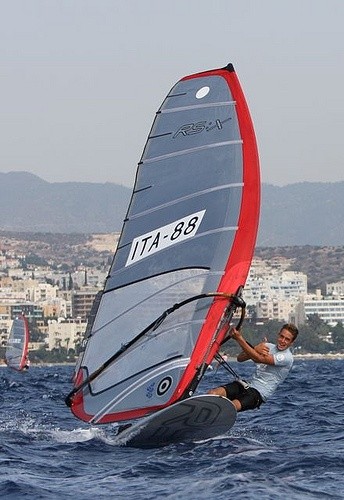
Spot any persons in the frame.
[208,324,299,413]
[22,358,30,370]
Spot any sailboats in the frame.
[64,63,261,449]
[3,314,28,388]
